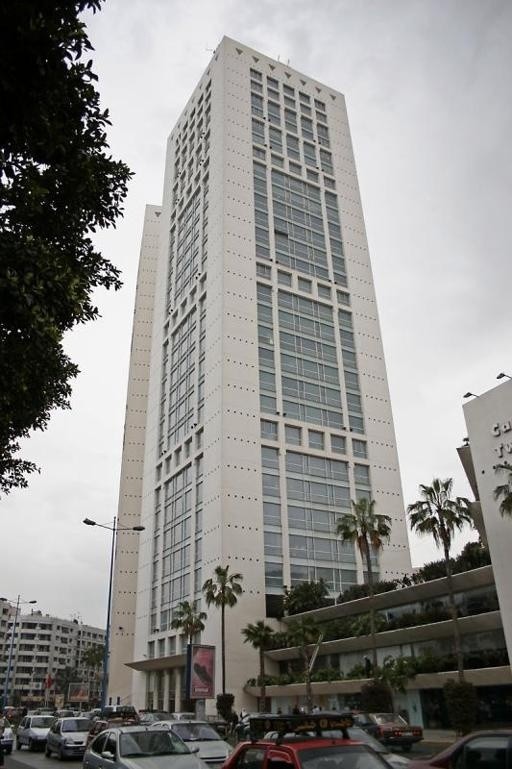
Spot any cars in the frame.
[0,736,6,765]
[217,713,400,769]
[44,717,94,762]
[0,705,229,728]
[0,716,15,756]
[148,720,235,769]
[404,729,511,769]
[351,713,424,753]
[261,723,411,769]
[16,715,54,753]
[81,726,212,769]
[87,720,137,748]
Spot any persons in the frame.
[276,703,365,715]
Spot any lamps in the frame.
[464,392,479,399]
[496,372,512,380]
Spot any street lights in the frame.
[82,515,147,703]
[0,592,36,708]
